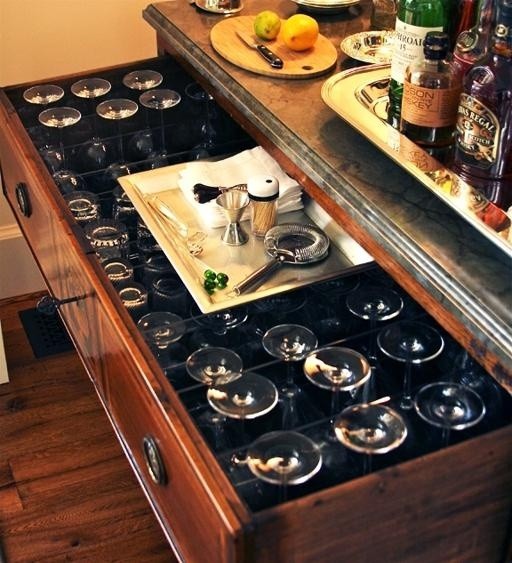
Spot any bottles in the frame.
[389,0,512,209]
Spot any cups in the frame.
[59,184,196,328]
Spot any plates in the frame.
[339,29,397,64]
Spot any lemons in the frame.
[254,10,281,41]
[281,13,319,51]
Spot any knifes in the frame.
[234,27,285,70]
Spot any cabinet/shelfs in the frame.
[2,0,511,562]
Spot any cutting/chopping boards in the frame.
[209,14,338,80]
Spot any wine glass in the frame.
[375,322,448,397]
[245,432,324,502]
[343,279,403,328]
[261,324,318,360]
[411,383,488,452]
[19,61,220,192]
[300,346,372,403]
[184,347,244,385]
[193,308,249,337]
[206,373,280,435]
[330,402,410,476]
[138,315,185,351]
